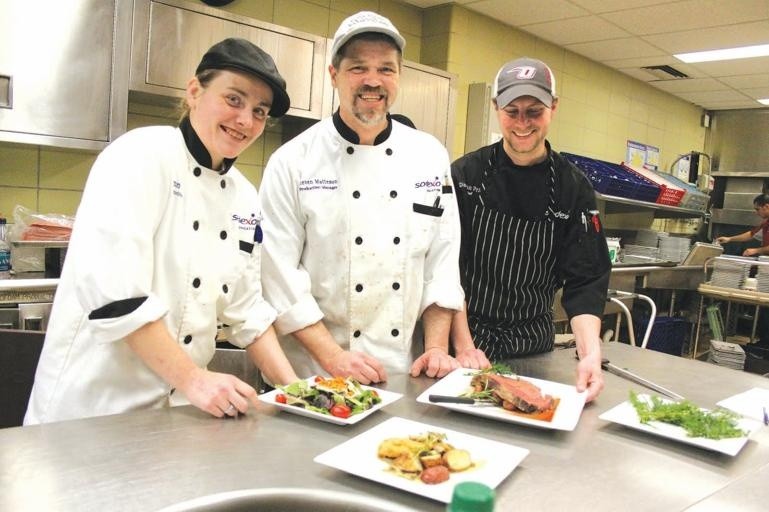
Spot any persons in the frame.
[717,193,769,257]
[19,37,303,426]
[256,9,464,386]
[447,56,613,404]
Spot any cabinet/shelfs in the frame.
[594,190,713,274]
[464,83,503,153]
[129,0,326,122]
[708,172,769,244]
[0,0,132,151]
[0,302,54,332]
[320,38,459,149]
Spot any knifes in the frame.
[429,394,497,407]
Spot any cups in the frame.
[605,238,620,262]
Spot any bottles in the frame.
[0,217,11,280]
[445,477,497,510]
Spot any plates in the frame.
[598,392,763,461]
[413,365,590,434]
[246,375,405,425]
[311,414,532,506]
[718,385,769,426]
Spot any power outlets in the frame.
[679,158,690,178]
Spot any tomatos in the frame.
[330,404,352,418]
[275,394,286,404]
[315,376,324,382]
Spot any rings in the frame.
[223,405,233,413]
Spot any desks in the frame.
[1,337,768,512]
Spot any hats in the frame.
[196,38,290,117]
[492,58,556,109]
[331,11,406,62]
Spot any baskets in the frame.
[622,317,689,356]
[560,152,710,212]
